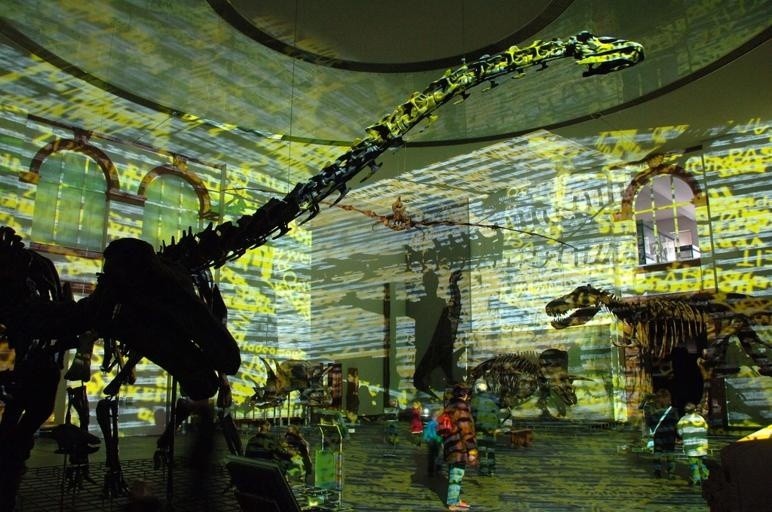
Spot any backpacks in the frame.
[436,412,452,437]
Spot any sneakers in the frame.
[448,499,471,512]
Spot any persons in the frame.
[643,386,679,479]
[245,421,293,481]
[282,420,313,479]
[473,389,501,478]
[676,401,712,487]
[422,409,443,474]
[435,384,477,512]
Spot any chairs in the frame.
[227,454,302,512]
